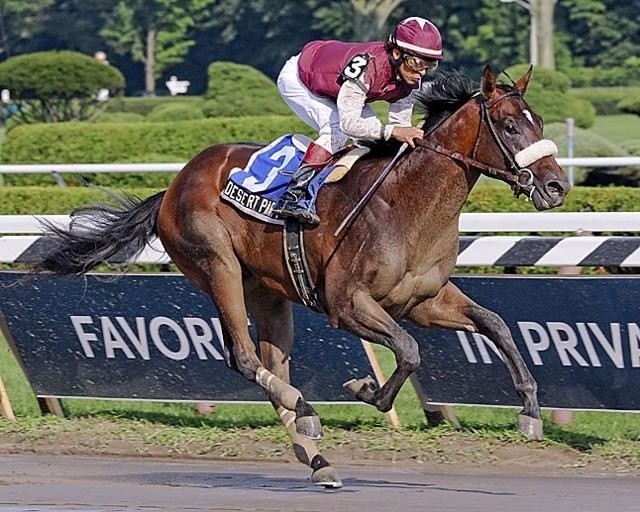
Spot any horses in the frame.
[22,64,571,488]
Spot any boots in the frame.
[270,141,337,225]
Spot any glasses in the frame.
[404,56,439,73]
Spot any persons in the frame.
[275,16,443,226]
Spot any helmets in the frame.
[386,15,445,61]
